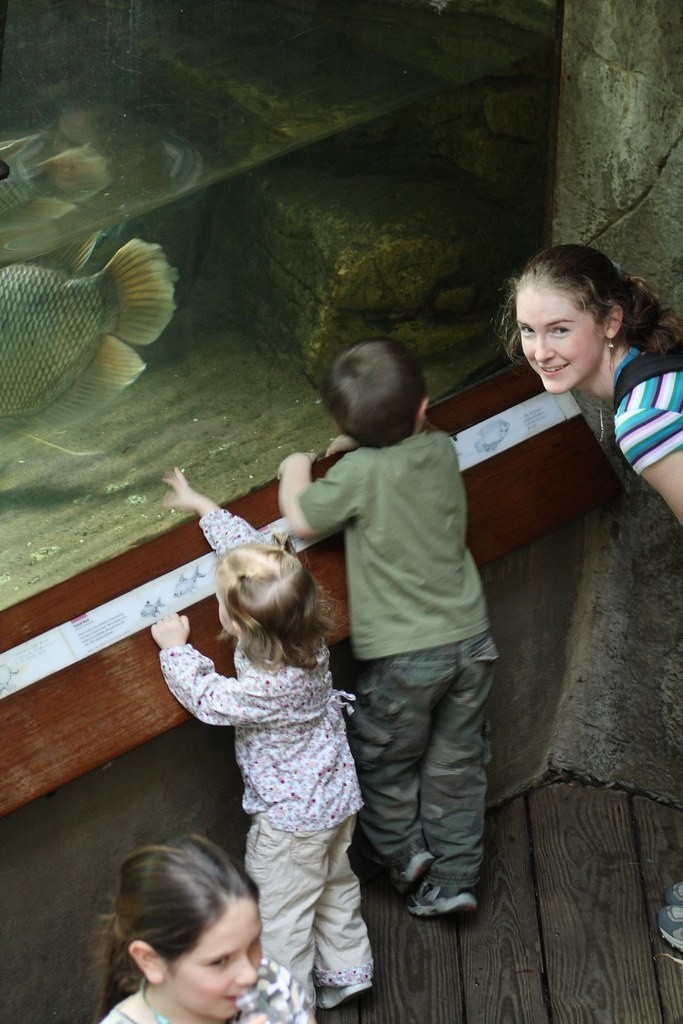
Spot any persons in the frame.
[277,335,499,917]
[91,831,318,1024]
[497,240,683,953]
[150,464,374,1024]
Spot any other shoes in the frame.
[657,880,683,952]
[316,980,373,1009]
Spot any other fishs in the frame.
[1,228,179,455]
[0,132,108,221]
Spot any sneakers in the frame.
[389,849,436,894]
[406,880,478,917]
